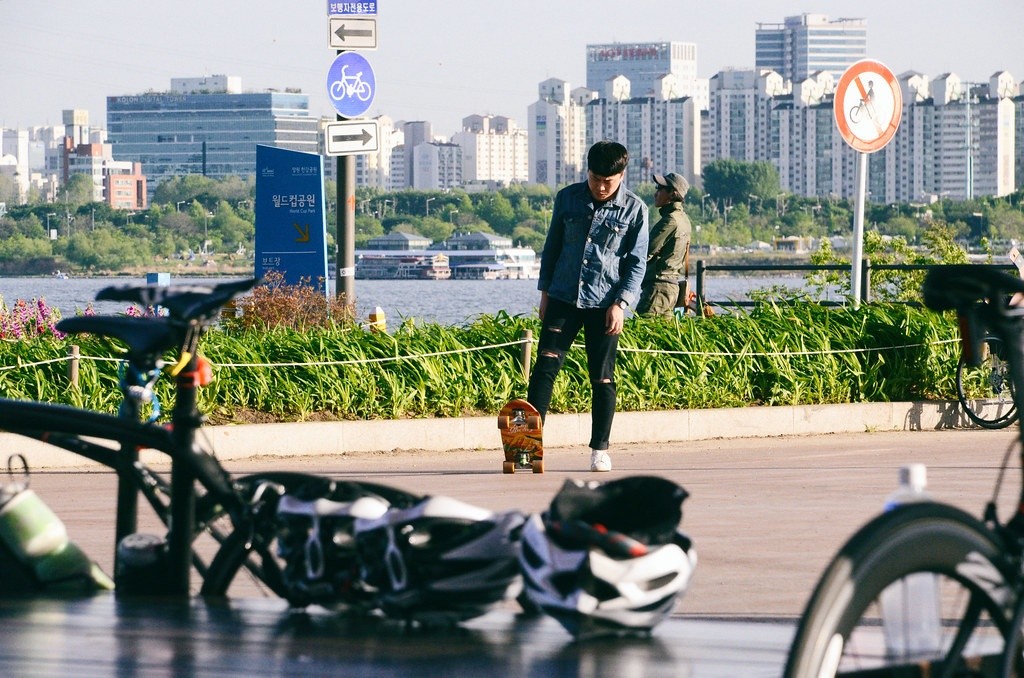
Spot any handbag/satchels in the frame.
[675,280,691,307]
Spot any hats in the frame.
[653,173,689,199]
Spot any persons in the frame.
[526,140,649,473]
[632,173,691,317]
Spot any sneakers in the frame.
[590,449,611,472]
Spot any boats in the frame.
[398,254,451,280]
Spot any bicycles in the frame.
[1,276,528,622]
[780,414,1024,678]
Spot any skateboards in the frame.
[494,399,545,475]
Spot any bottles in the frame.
[880,463,944,657]
[0,453,115,595]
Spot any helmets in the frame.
[516,475,700,644]
[234,470,525,627]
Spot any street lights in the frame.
[47,214,56,238]
[205,211,213,238]
[450,209,458,223]
[427,198,435,216]
[93,208,96,232]
[178,201,185,213]
